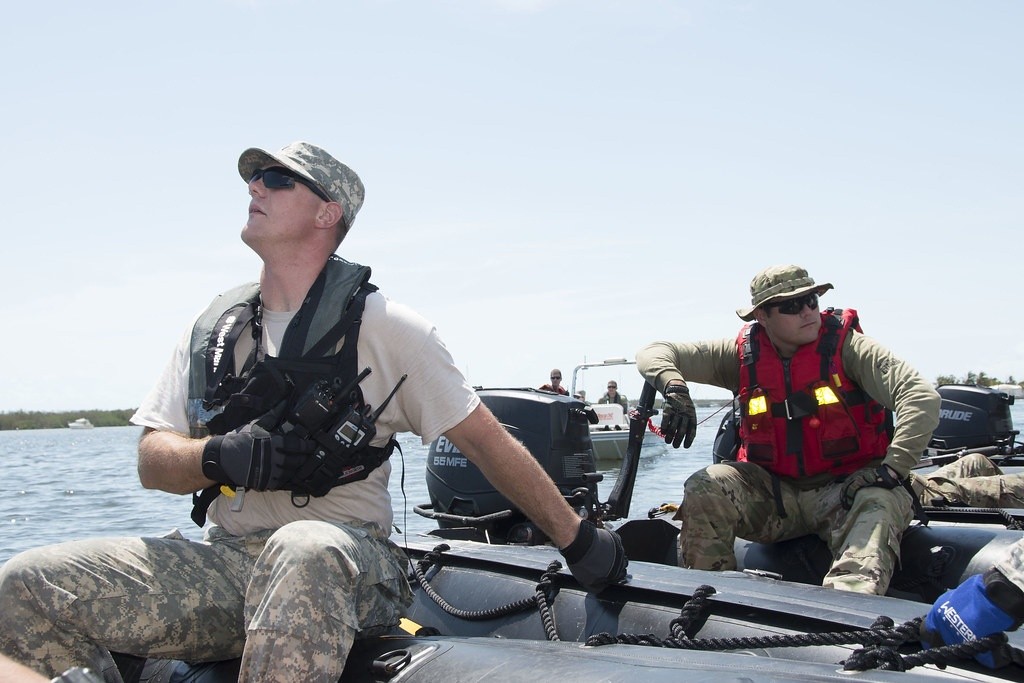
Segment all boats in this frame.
[68,418,94,430]
[568,356,670,462]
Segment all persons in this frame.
[597,380,631,417]
[633,265,943,597]
[0,140,628,682]
[538,368,570,397]
[876,451,1024,512]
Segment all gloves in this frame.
[839,464,902,511]
[558,519,628,593]
[661,385,697,449]
[918,567,1024,670]
[201,400,321,493]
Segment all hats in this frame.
[238,140,365,233]
[736,264,834,322]
[573,390,585,396]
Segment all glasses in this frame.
[551,376,560,379]
[766,291,819,315]
[249,166,331,203]
[574,395,584,399]
[608,386,616,388]
